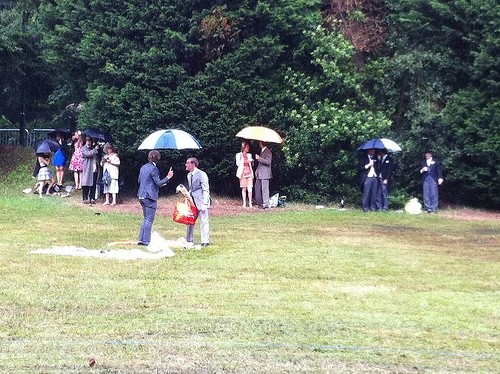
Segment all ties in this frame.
[379,158,382,166]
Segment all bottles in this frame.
[341,198,344,208]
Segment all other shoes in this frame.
[201,243,208,246]
[138,240,148,246]
[83,199,89,204]
[90,200,96,204]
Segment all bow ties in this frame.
[370,157,373,160]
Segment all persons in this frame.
[34,137,63,154]
[357,148,381,212]
[136,149,174,246]
[184,158,211,247]
[235,141,255,209]
[33,129,107,205]
[377,149,393,211]
[419,149,444,214]
[100,142,121,206]
[253,139,273,210]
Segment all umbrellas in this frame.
[137,127,203,172]
[356,138,403,158]
[234,126,284,158]
[80,128,116,149]
[48,127,74,139]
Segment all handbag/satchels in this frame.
[102,169,111,186]
[173,195,197,224]
[279,196,287,207]
